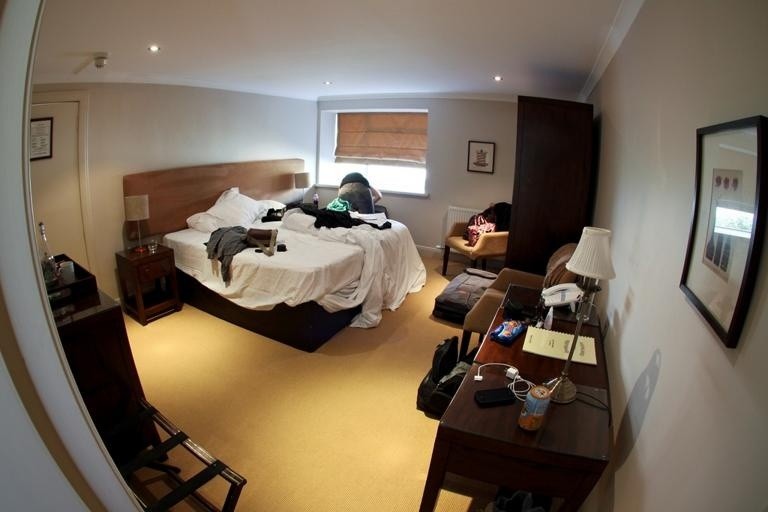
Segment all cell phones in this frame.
[474,386,516,409]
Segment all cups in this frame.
[59,261,75,284]
[148,240,157,251]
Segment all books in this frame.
[521,325,598,368]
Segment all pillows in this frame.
[257,200,286,211]
[186,186,270,233]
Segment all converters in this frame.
[507,367,520,379]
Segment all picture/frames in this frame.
[30,117,54,161]
[466,140,496,174]
[679,114,768,349]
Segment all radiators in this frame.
[445,205,485,247]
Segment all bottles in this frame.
[542,305,553,329]
[38,222,59,286]
[313,192,321,209]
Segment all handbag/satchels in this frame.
[417,336,479,419]
[467,223,496,246]
[247,229,278,249]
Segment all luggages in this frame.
[433,268,498,324]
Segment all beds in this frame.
[122,157,427,352]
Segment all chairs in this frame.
[460,242,581,365]
[442,201,509,278]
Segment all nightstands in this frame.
[115,243,181,327]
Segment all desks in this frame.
[40,253,248,512]
[419,285,615,512]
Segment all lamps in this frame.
[294,172,311,207]
[544,212,618,405]
[124,194,151,253]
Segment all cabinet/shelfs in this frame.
[507,91,598,275]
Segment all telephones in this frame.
[541,283,584,307]
